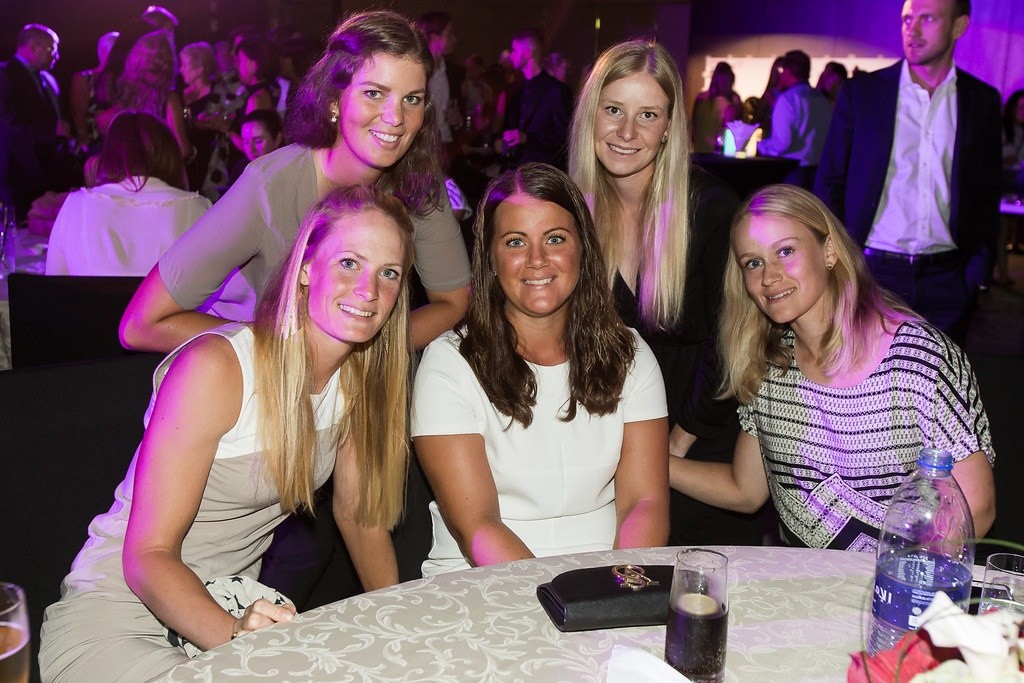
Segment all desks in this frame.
[693,150,801,196]
[140,547,1024,683]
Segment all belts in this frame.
[862,247,958,265]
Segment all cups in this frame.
[0,582,31,683]
[976,553,1024,616]
[664,547,728,682]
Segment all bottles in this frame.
[867,448,974,651]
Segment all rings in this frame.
[231,632,237,639]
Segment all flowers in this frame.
[848,590,1024,683]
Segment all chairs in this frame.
[7,271,146,370]
[0,352,166,683]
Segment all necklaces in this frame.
[319,149,335,192]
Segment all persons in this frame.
[0,0,872,319]
[38,183,410,683]
[669,184,997,559]
[410,162,669,583]
[815,0,1002,348]
[117,13,475,616]
[563,37,777,546]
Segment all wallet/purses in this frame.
[536,565,716,632]
